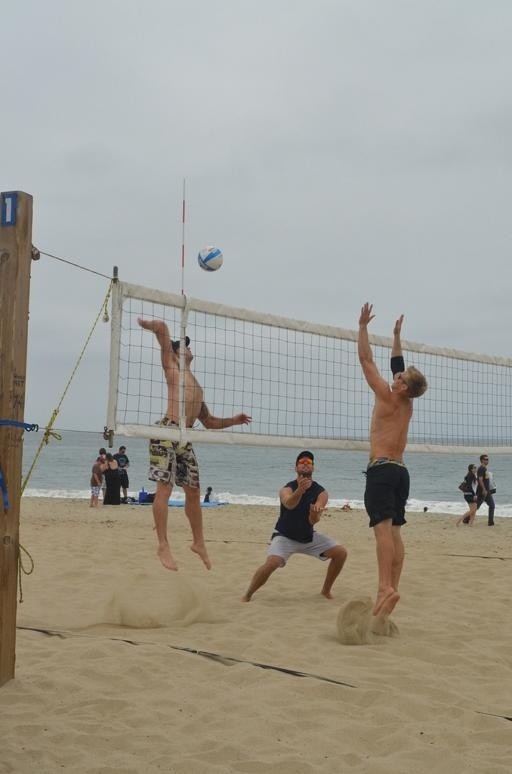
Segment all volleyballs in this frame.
[196,245,223,271]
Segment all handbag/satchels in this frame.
[458,479,472,492]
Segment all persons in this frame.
[454,462,479,528]
[462,454,498,528]
[90,456,106,507]
[240,450,348,603]
[357,303,430,629]
[204,486,217,503]
[103,453,121,506]
[99,448,108,499]
[137,314,254,572]
[112,445,129,503]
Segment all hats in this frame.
[296,451,314,466]
[171,336,190,351]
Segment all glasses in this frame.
[483,458,489,461]
[297,458,313,464]
[398,372,410,390]
